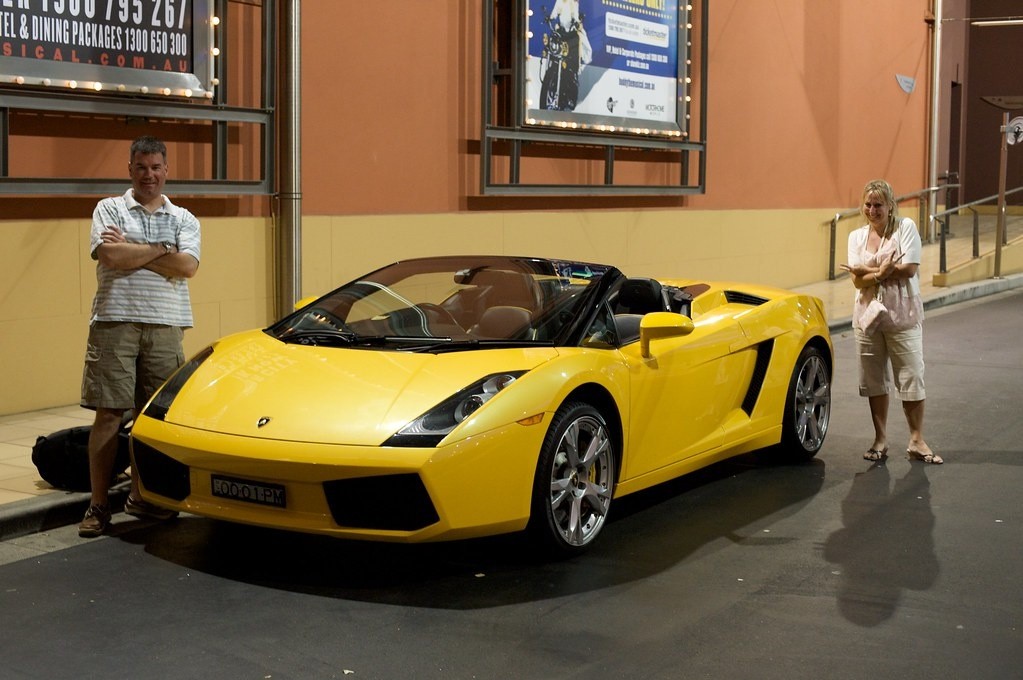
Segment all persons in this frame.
[839,180,943,465]
[77,135,202,537]
[546,0,592,75]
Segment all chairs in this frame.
[463,278,665,345]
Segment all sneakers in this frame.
[125,496,179,520]
[78,504,112,536]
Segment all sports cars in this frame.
[128,253,837,558]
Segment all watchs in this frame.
[160,241,172,255]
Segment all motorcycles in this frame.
[538,4,587,113]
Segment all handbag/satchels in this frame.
[859,284,888,336]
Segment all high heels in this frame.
[863,447,888,461]
[908,449,943,464]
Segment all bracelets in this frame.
[874,272,882,283]
